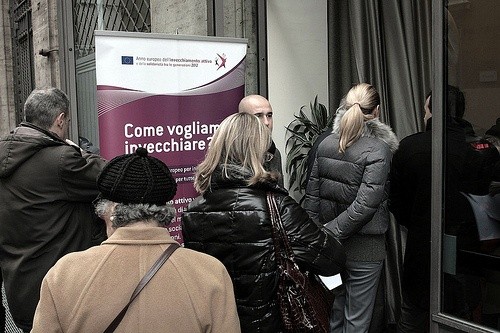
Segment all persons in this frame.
[180,112,347,333]
[475,117,500,196]
[238,95,284,188]
[0,85,111,333]
[306,97,387,333]
[29,147,241,333]
[390,85,493,333]
[304,83,400,333]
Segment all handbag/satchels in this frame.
[464,191,500,240]
[266,191,335,333]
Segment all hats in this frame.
[92,147,177,205]
